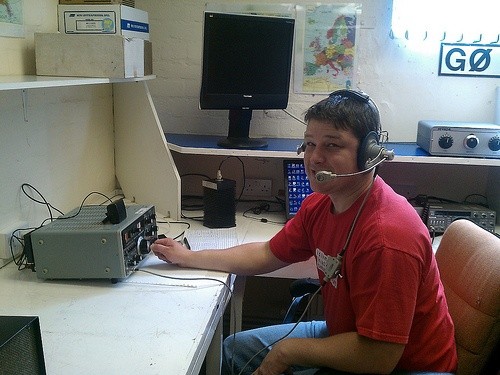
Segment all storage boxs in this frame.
[34,4,151,79]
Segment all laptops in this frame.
[283,160,314,220]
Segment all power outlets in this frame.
[242,177,272,197]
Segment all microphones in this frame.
[316,158,386,183]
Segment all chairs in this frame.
[281,217,500,375]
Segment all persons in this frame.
[150,90,459,375]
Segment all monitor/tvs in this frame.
[197,10,295,148]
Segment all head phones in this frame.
[295,90,395,175]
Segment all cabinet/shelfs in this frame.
[0,73,500,375]
